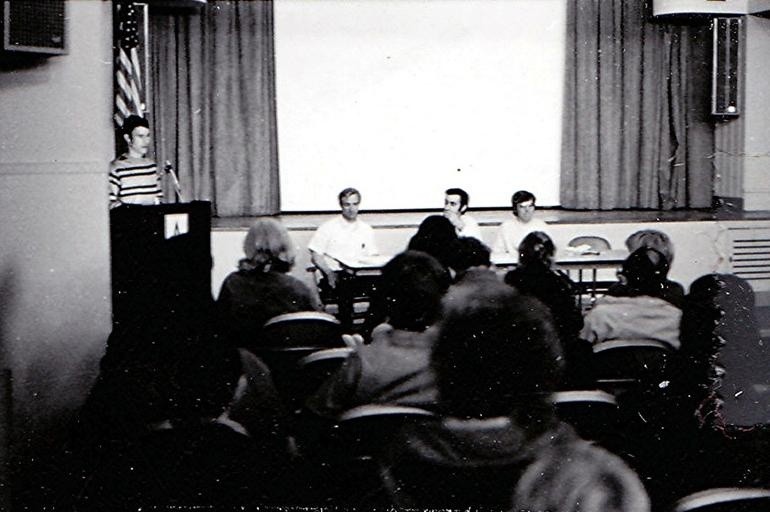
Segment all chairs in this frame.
[260,312,355,401]
[307,267,370,321]
[593,339,675,385]
[568,236,612,313]
[334,406,440,460]
[407,454,534,511]
[546,390,619,440]
[672,486,770,512]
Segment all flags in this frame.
[112,0,147,156]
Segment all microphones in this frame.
[163,159,182,193]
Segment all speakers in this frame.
[0,1,68,57]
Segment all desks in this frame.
[336,250,628,334]
